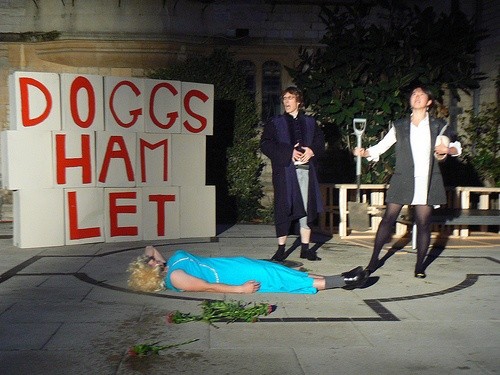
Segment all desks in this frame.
[381,206,500,227]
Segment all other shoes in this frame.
[300,249,321,261]
[271,250,286,262]
[340,271,369,290]
[415,272,425,278]
[364,259,380,271]
[341,266,363,279]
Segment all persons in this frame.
[259,87,326,261]
[354,85,463,279]
[126,244,369,295]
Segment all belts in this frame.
[302,162,309,165]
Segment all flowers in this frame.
[164,299,272,330]
[126,338,199,359]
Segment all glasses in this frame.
[283,96,296,100]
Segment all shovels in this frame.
[348,119,369,231]
